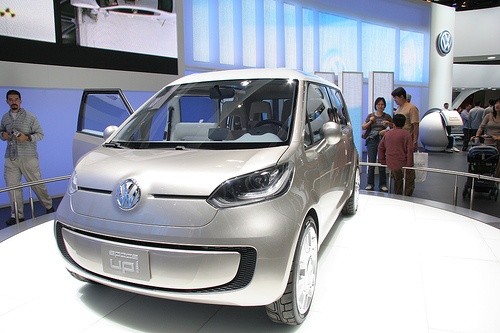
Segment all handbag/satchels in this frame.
[361,112,374,139]
[413,148,428,183]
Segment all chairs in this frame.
[221,98,329,146]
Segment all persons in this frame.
[444,103,463,114]
[381,87,419,152]
[0,90,54,226]
[472,98,500,148]
[461,103,473,154]
[482,99,497,120]
[362,97,393,192]
[468,99,484,144]
[377,114,416,196]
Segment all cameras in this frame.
[10,128,20,137]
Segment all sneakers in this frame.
[381,185,388,191]
[364,184,373,190]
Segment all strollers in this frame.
[462,135,500,201]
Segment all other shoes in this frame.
[45,205,54,214]
[6,217,26,226]
[442,147,452,153]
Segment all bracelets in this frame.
[25,134,32,141]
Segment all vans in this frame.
[54,67,360,325]
